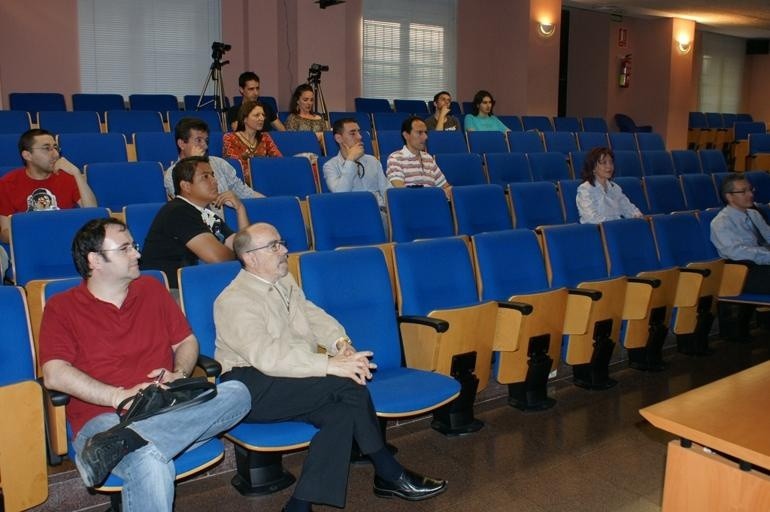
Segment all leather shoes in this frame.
[373,467,447,500]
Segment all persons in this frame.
[465,90,511,134]
[285,84,328,144]
[140,156,249,288]
[39,218,252,512]
[424,91,460,132]
[212,221,449,510]
[221,102,283,179]
[711,174,770,295]
[386,118,451,198]
[163,117,266,205]
[229,72,284,131]
[323,118,394,225]
[0,129,98,243]
[575,147,643,224]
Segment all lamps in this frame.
[537,23,555,38]
[677,41,690,55]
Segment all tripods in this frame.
[315,84,328,121]
[195,60,229,133]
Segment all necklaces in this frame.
[239,132,257,149]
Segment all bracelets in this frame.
[174,369,192,379]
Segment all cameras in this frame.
[308,63,329,84]
[211,42,232,59]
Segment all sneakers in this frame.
[75,433,126,488]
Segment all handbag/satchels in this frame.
[121,377,217,422]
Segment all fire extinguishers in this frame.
[619,52,633,88]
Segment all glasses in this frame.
[104,243,140,252]
[31,145,59,152]
[725,186,755,193]
[245,239,287,253]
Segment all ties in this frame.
[745,213,770,249]
